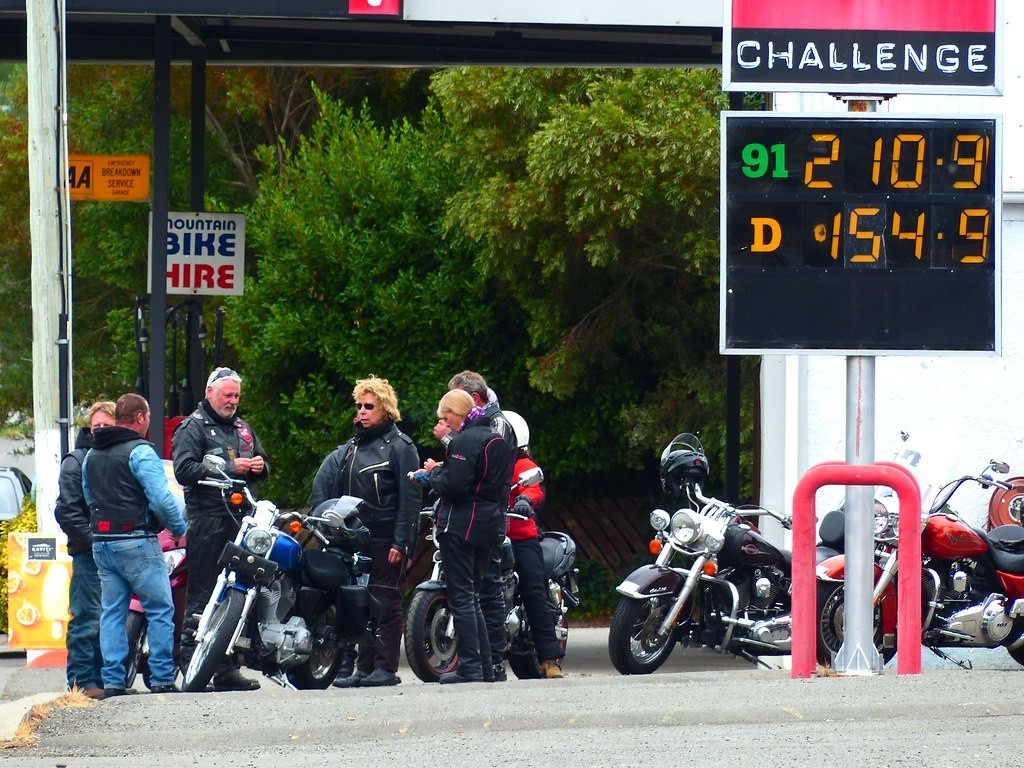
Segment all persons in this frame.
[81,393,188,695]
[170,366,274,691]
[409,368,566,682]
[53,400,117,700]
[303,373,421,686]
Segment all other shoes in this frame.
[205,681,213,692]
[539,657,564,678]
[493,661,507,681]
[214,669,261,693]
[438,667,483,685]
[151,684,182,694]
[484,675,495,682]
[72,684,106,700]
[102,688,139,696]
[333,668,368,689]
[359,666,401,687]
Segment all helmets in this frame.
[311,494,372,551]
[502,409,530,447]
[659,450,709,498]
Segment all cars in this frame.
[0,467,32,523]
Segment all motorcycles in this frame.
[608,430,1024,675]
[125,454,382,693]
[406,467,580,685]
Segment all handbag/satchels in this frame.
[216,540,281,590]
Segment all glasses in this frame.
[212,369,241,383]
[354,402,380,410]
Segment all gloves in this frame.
[513,494,534,517]
